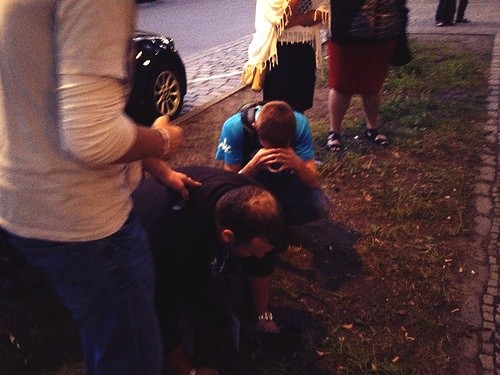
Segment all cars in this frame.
[125,31,187,124]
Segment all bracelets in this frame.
[153,127,170,159]
[258,312,273,320]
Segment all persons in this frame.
[436,0,456,27]
[248,0,331,114]
[0,0,202,375]
[137,165,280,375]
[214,100,331,226]
[326,0,404,152]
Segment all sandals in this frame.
[326,132,343,152]
[364,128,391,147]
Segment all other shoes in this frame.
[437,20,470,27]
